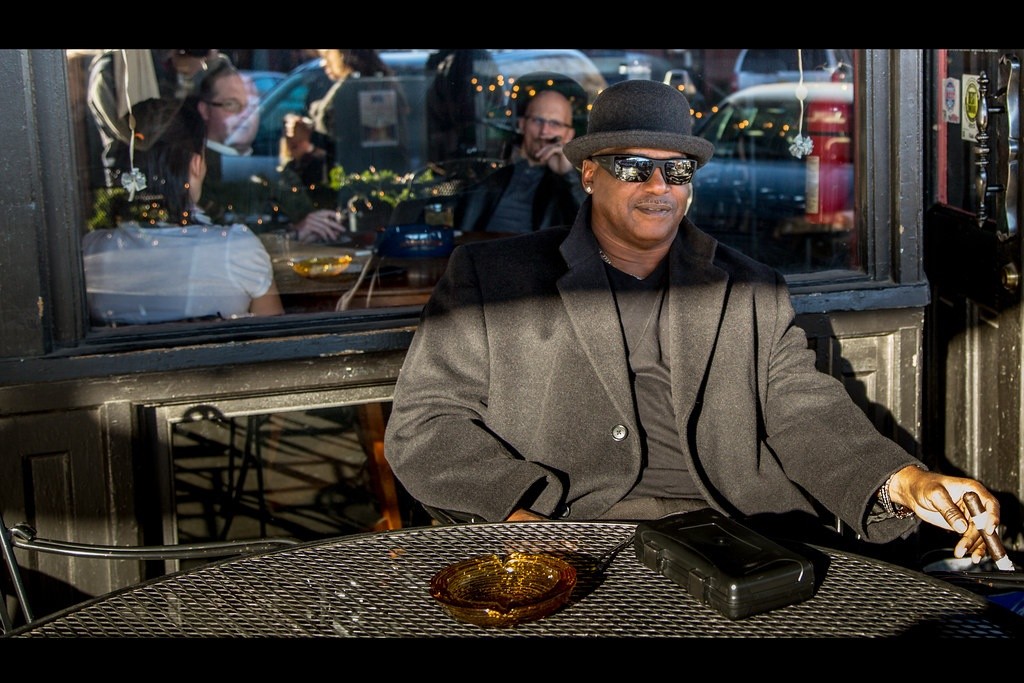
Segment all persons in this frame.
[456,90,592,251]
[81,98,288,325]
[380,79,1002,565]
[197,69,348,245]
[89,45,509,227]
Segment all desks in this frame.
[0,234,1024,683]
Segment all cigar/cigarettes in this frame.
[548,135,561,144]
[963,492,1015,571]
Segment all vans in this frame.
[726,47,855,100]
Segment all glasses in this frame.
[529,116,572,129]
[207,99,264,115]
[586,154,697,185]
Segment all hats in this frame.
[562,80,715,170]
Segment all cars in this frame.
[592,50,710,117]
[210,67,315,152]
[209,49,612,224]
[686,80,855,261]
